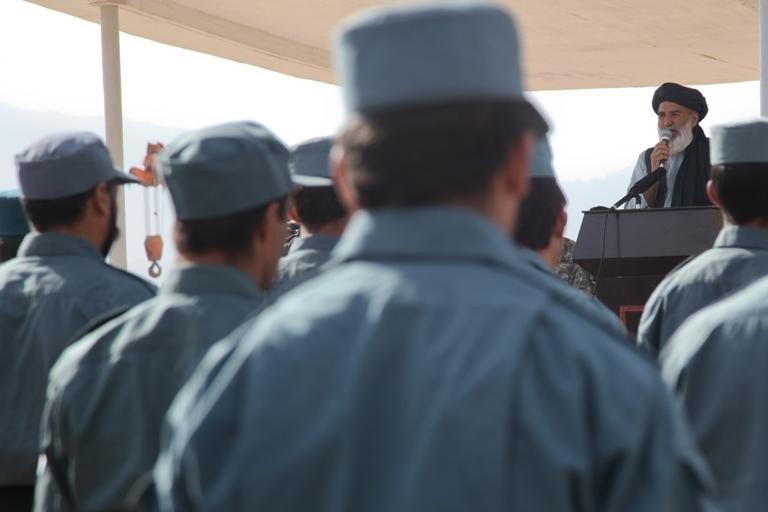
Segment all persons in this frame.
[148,0,719,511]
[507,113,569,275]
[268,133,345,303]
[34,119,305,511]
[623,82,713,207]
[0,124,161,510]
[635,117,767,448]
[0,187,30,266]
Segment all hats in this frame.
[159,120,333,220]
[652,83,708,122]
[290,137,333,181]
[530,134,554,176]
[341,6,550,135]
[1,190,30,236]
[14,131,142,201]
[709,121,768,166]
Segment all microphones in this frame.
[659,129,673,167]
[609,168,666,212]
